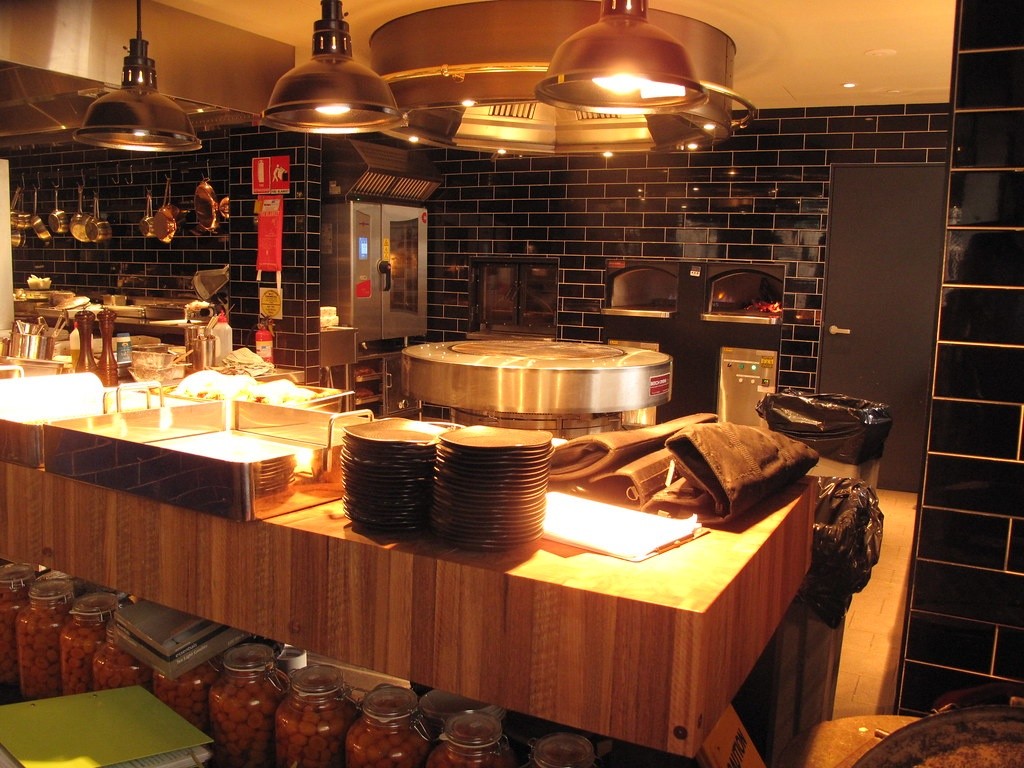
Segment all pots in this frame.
[9,187,31,249]
[48,186,69,233]
[31,188,52,242]
[142,179,181,243]
[194,178,221,234]
[69,188,112,242]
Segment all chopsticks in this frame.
[207,314,220,329]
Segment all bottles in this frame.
[70,307,118,386]
[427,710,595,768]
[274,665,354,768]
[209,644,290,768]
[345,683,434,768]
[60,594,217,729]
[213,311,233,367]
[0,563,74,701]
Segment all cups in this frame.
[185,327,215,369]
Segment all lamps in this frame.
[72,1,203,152]
[533,1,709,114]
[261,0,402,134]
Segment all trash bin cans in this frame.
[732,476,873,768]
[762,391,892,496]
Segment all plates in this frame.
[340,418,448,530]
[431,425,554,551]
[420,689,508,722]
[258,454,296,491]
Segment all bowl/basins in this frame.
[27,280,73,307]
[103,295,127,306]
[129,344,192,385]
